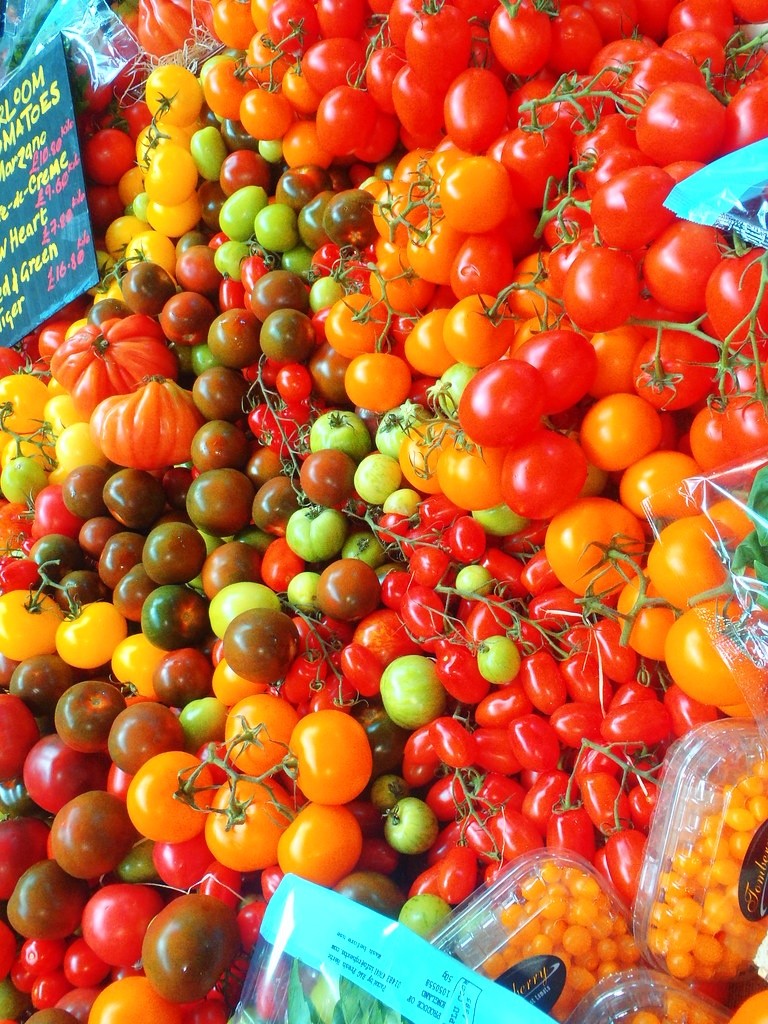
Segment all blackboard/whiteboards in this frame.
[0,30,101,349]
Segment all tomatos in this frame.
[2,0,768,1024]
